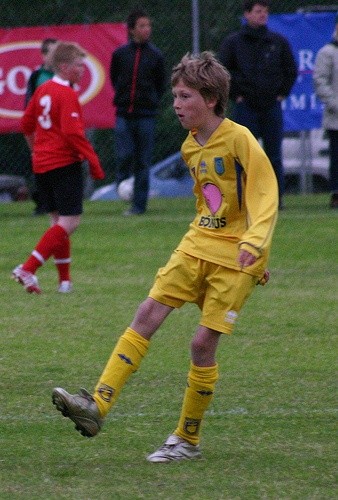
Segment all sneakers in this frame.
[147,433,203,465]
[12,264,43,295]
[52,386,102,438]
[56,281,75,296]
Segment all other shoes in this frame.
[122,207,142,216]
[331,194,338,209]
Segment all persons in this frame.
[11,40,105,294]
[50,51,280,466]
[312,13,338,211]
[215,0,300,213]
[108,9,174,221]
[24,37,61,216]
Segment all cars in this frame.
[90,137,332,198]
